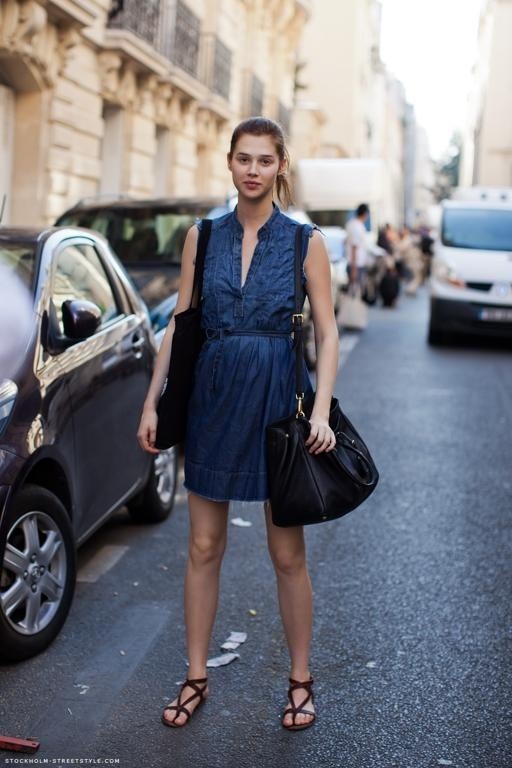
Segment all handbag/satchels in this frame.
[264,391,379,528]
[152,218,214,452]
[335,293,369,331]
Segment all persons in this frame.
[136,117,339,733]
[344,203,439,307]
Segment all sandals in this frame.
[161,677,209,727]
[280,674,318,732]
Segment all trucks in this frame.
[283,150,399,293]
[417,184,509,350]
